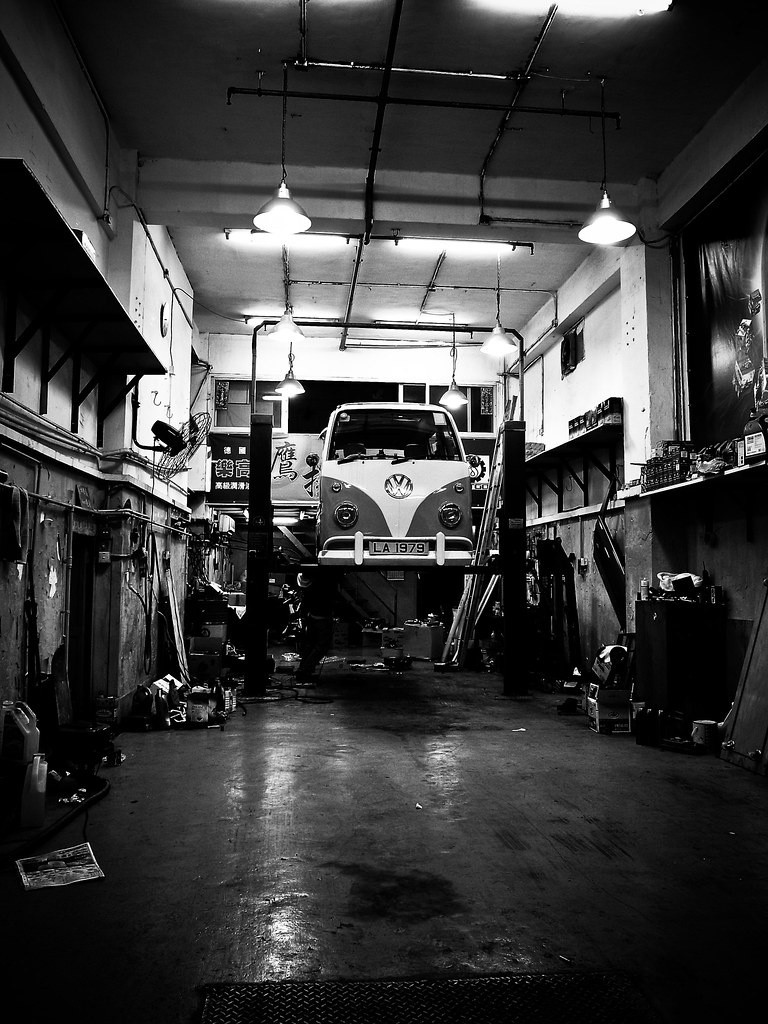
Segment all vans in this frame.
[306,402,475,569]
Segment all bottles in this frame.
[641,578,649,601]
[213,677,225,713]
[643,447,698,492]
[0,700,40,763]
[744,412,766,463]
[20,753,48,828]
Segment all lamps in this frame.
[267,245,306,343]
[439,313,469,410]
[481,260,517,352]
[275,342,305,394]
[253,69,312,234]
[578,85,637,245]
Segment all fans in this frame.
[131,412,213,481]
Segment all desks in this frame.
[0,774,111,848]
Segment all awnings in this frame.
[0,157,168,419]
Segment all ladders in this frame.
[451,394,518,667]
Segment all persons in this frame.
[297,568,341,688]
